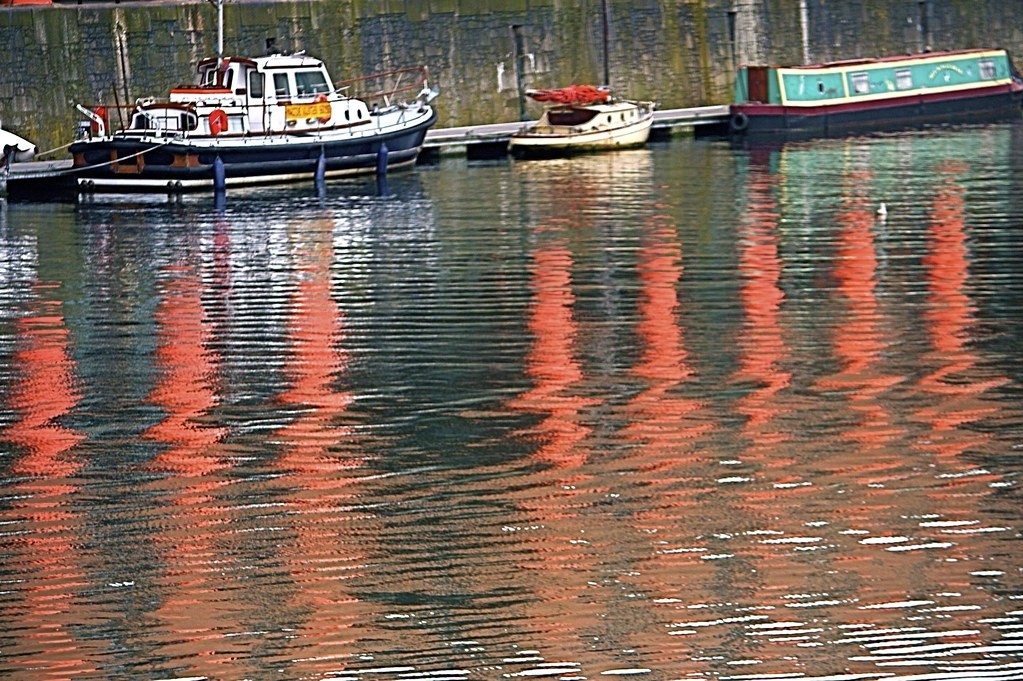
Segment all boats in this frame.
[729,46,1023,136]
[67,0,437,194]
[506,99,656,157]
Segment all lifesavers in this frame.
[314,94,332,123]
[93,106,109,132]
[731,113,749,130]
[209,109,228,136]
